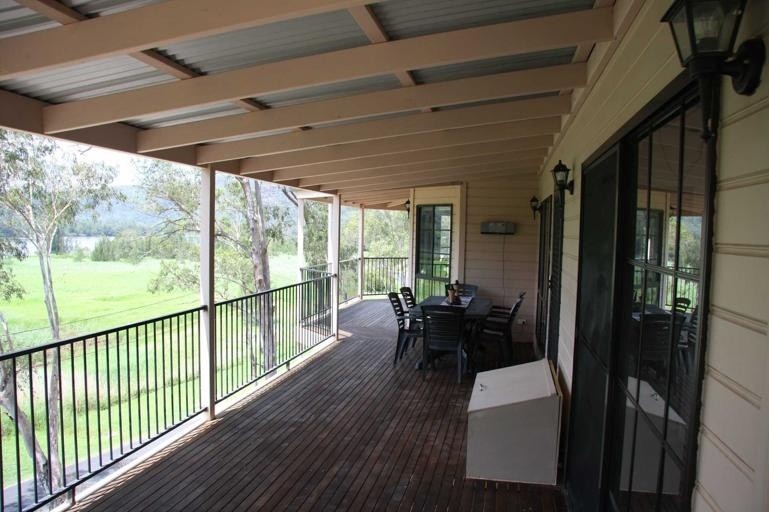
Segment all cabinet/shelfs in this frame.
[620,376,688,497]
[465,356,564,487]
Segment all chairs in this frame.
[420,305,470,384]
[491,289,526,320]
[445,283,476,298]
[470,297,523,376]
[400,287,426,346]
[388,292,426,365]
[627,286,697,400]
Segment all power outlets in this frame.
[517,319,527,327]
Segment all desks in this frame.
[408,295,493,372]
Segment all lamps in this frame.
[530,195,543,220]
[480,222,515,235]
[660,1,766,139]
[550,159,574,207]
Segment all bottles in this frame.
[448,280,460,303]
